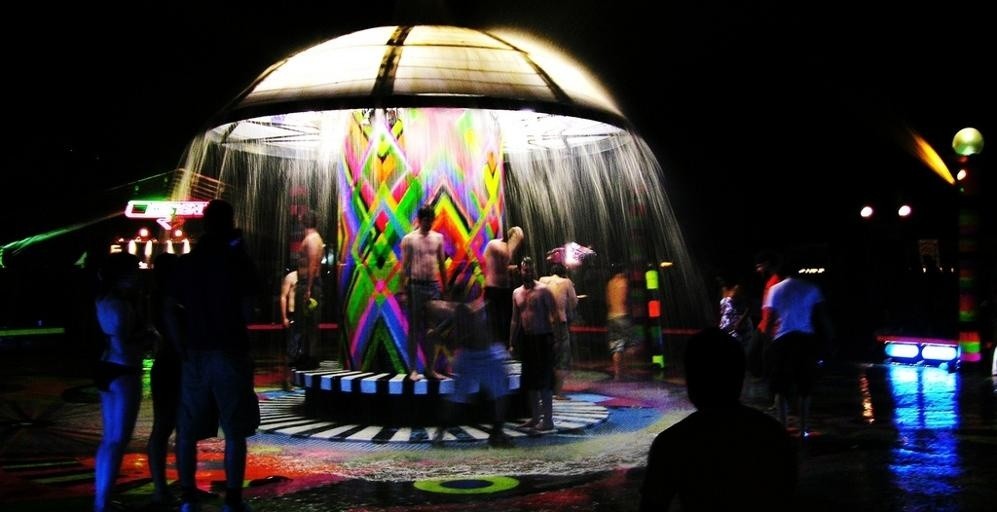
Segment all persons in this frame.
[606,258,645,387]
[540,259,578,396]
[84,249,149,512]
[297,208,324,372]
[508,255,565,439]
[481,225,524,344]
[637,258,834,510]
[395,204,447,385]
[172,198,262,512]
[425,285,514,444]
[138,250,223,512]
[279,267,300,394]
